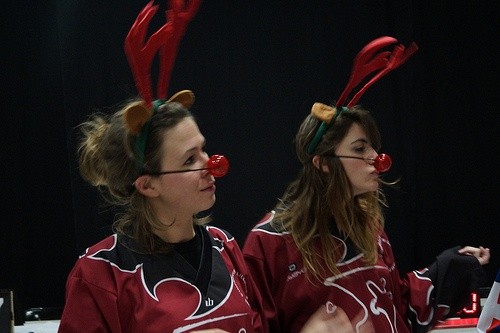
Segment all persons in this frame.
[242,100,491,333]
[57,90,355,333]
